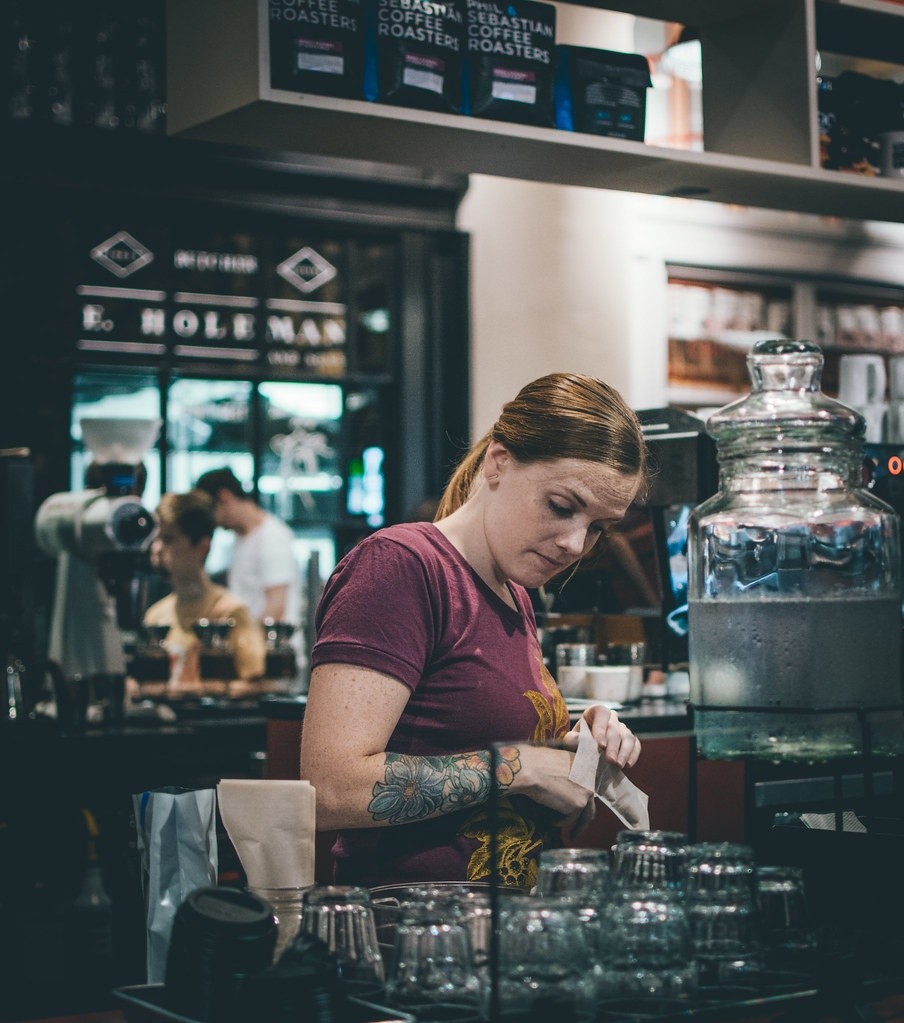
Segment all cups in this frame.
[758,865,811,950]
[390,885,492,992]
[166,886,279,1023]
[298,886,387,995]
[555,642,596,698]
[497,830,759,998]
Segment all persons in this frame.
[543,532,661,645]
[190,469,308,667]
[302,374,647,886]
[123,491,267,711]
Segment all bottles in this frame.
[261,621,298,681]
[134,627,169,685]
[686,339,903,764]
[190,618,235,684]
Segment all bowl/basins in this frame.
[585,665,642,700]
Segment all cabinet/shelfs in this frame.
[166,0,904,224]
[665,262,904,511]
